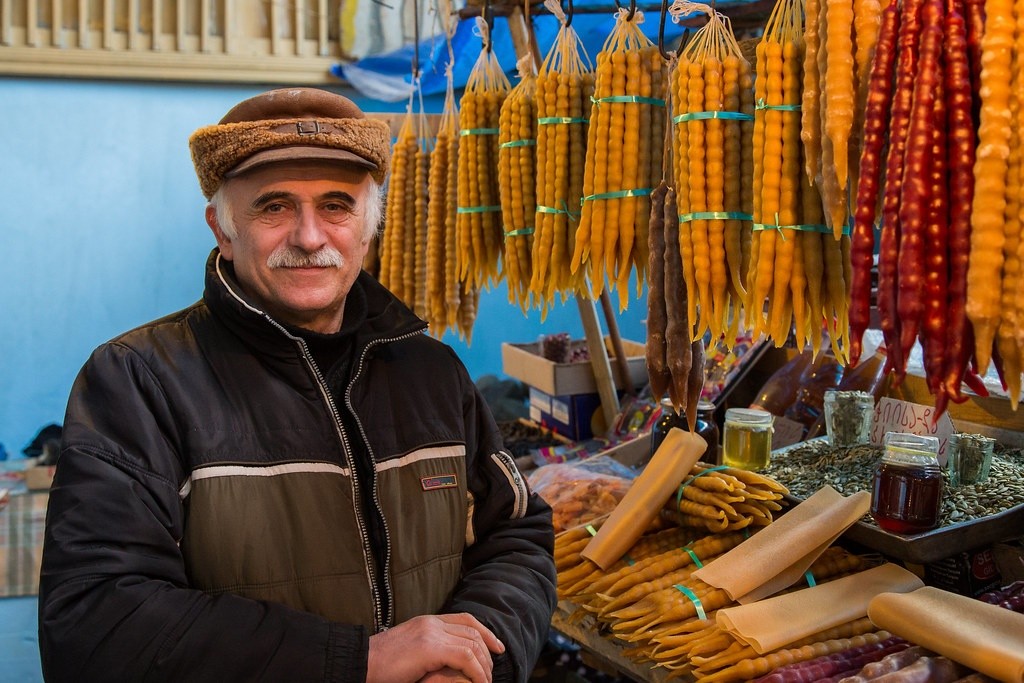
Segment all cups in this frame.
[948,434,994,486]
[824,395,874,447]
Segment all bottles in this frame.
[870,432,941,534]
[722,408,772,472]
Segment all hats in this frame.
[188,87,391,200]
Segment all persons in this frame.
[41,85,559,683]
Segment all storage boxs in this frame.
[0,439,57,600]
[502,336,1024,566]
